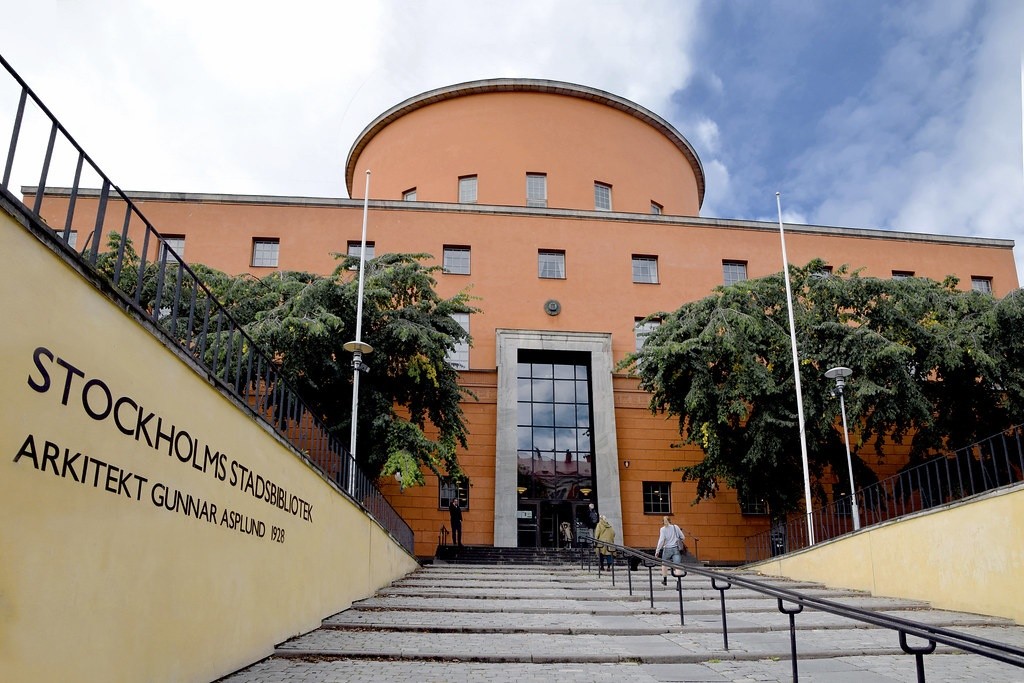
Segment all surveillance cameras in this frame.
[625,461,628,468]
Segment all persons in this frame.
[560,524,573,549]
[449,498,464,547]
[594,515,615,572]
[655,516,685,586]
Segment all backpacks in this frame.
[589,510,598,524]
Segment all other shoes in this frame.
[458,543,464,546]
[661,582,667,585]
[601,568,604,570]
[453,543,457,545]
[607,567,610,571]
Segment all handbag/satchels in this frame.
[593,537,599,548]
[673,524,686,555]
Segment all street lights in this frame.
[823,366,862,530]
[341,340,373,495]
